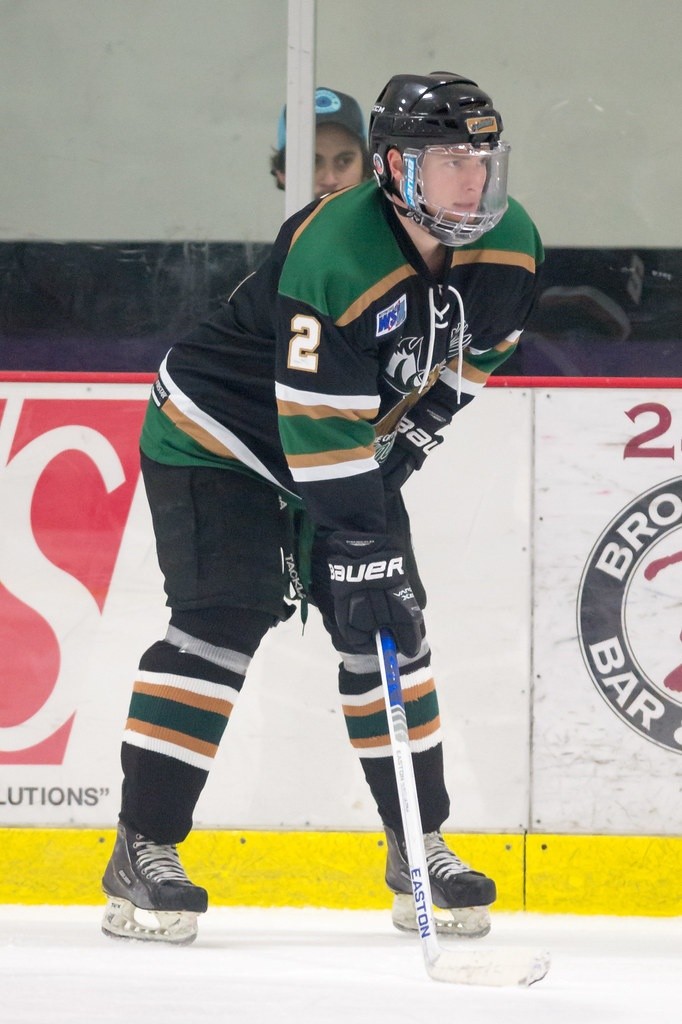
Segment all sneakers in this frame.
[382,824,497,939]
[101,820,208,945]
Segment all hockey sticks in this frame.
[375,625,556,986]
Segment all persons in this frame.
[265,91,368,197]
[103,72,557,944]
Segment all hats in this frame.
[277,87,364,150]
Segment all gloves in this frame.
[381,399,453,502]
[323,523,427,659]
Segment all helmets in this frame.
[368,70,511,247]
